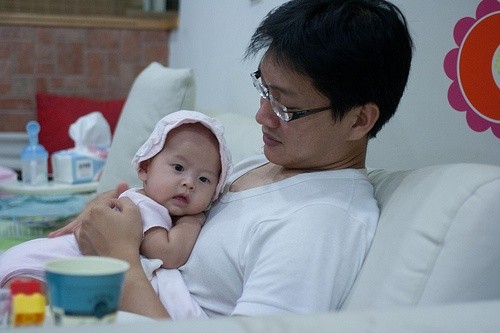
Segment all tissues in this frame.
[51,110,113,182]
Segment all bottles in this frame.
[19,122,48,187]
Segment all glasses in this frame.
[250,69,333,121]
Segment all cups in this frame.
[44,255,130,323]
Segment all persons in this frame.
[0,109,231,306]
[47,0,414,322]
